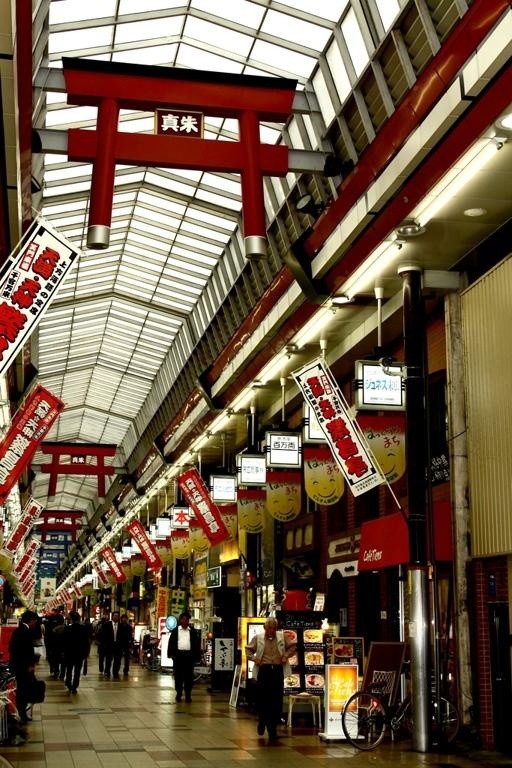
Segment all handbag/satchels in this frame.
[27,670,46,704]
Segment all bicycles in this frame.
[193,648,212,682]
[143,636,162,671]
[341,660,462,751]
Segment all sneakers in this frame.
[256,723,280,744]
[175,694,182,701]
[184,696,192,703]
[51,670,79,695]
[98,669,130,678]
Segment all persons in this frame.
[244,617,295,739]
[168,612,199,703]
[8,607,134,722]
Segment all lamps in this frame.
[296,193,325,220]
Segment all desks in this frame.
[287,695,322,729]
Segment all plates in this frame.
[297,694,313,697]
